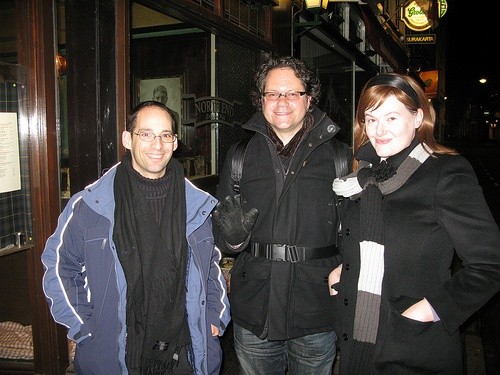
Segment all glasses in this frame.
[262,91,307,100]
[134,131,177,143]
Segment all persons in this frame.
[41,101,232,375]
[328,72,500,375]
[211,57,356,375]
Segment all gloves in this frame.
[213,194,260,246]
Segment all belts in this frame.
[245,242,339,263]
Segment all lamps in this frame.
[291,0,328,58]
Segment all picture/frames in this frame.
[135,74,186,147]
